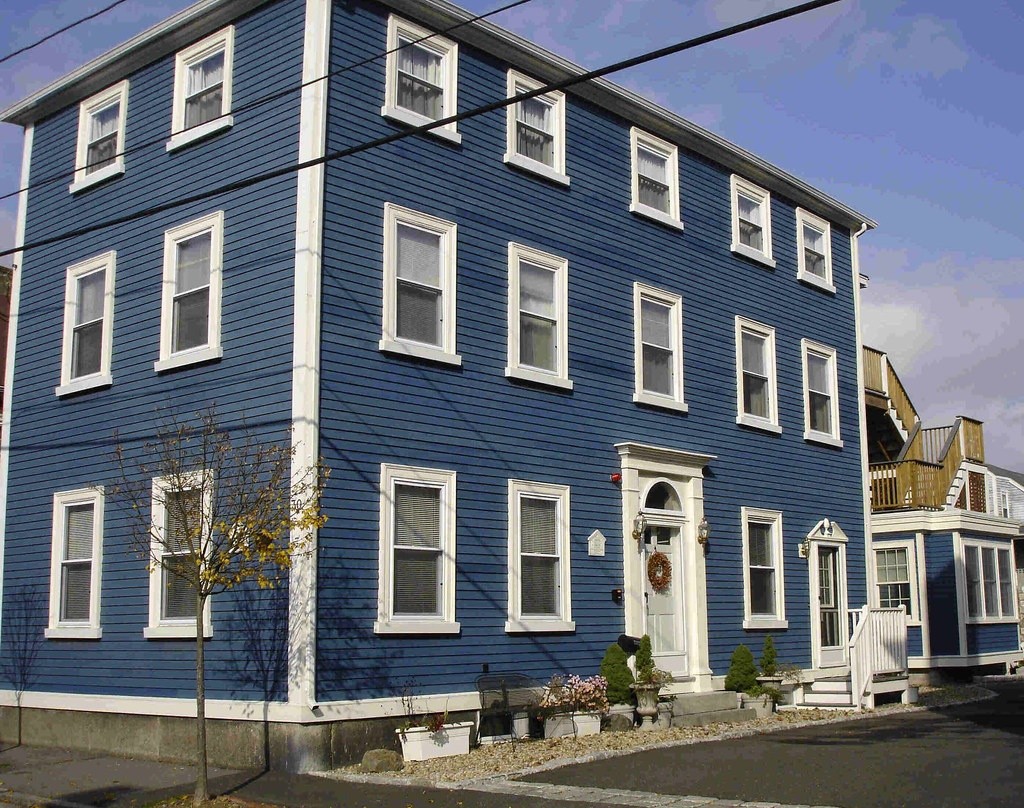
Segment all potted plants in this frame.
[630,634,679,732]
[599,643,638,730]
[724,636,801,718]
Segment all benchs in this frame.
[473,673,578,753]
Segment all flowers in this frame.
[649,551,673,589]
[534,671,609,722]
[394,676,453,742]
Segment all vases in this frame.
[395,721,474,762]
[544,709,602,739]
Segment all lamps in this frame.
[697,517,712,544]
[633,508,647,545]
[799,536,811,558]
